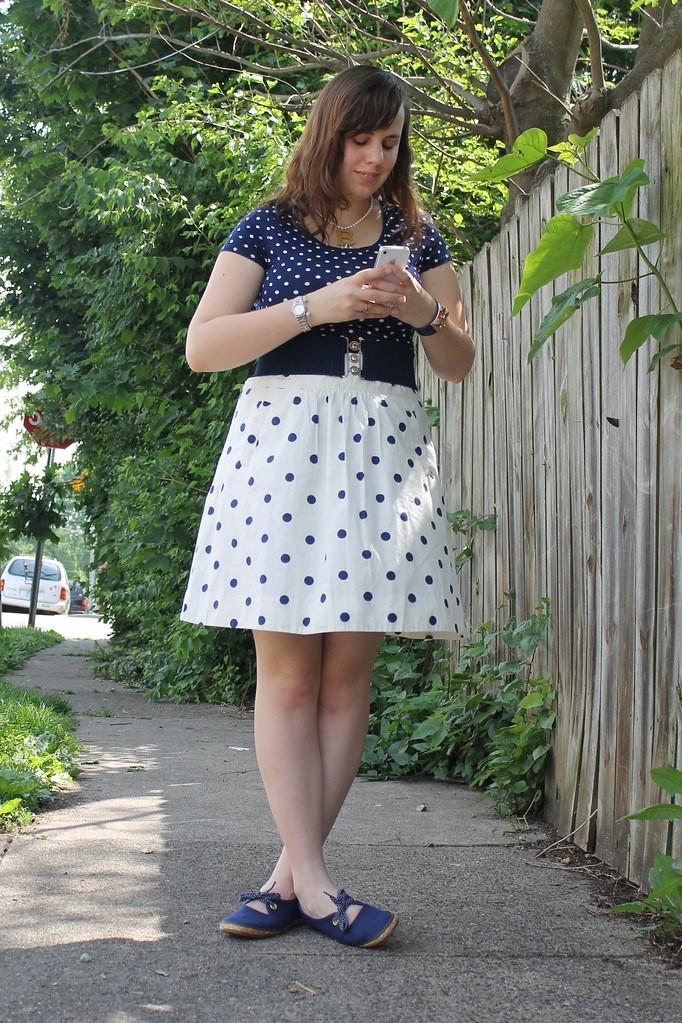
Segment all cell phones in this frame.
[375,245,410,269]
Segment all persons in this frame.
[181,65,475,947]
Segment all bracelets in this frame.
[414,301,449,336]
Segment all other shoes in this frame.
[297,886,398,947]
[220,881,306,936]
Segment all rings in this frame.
[363,302,367,313]
[390,303,395,308]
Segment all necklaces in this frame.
[314,196,373,246]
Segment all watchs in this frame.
[292,296,311,333]
